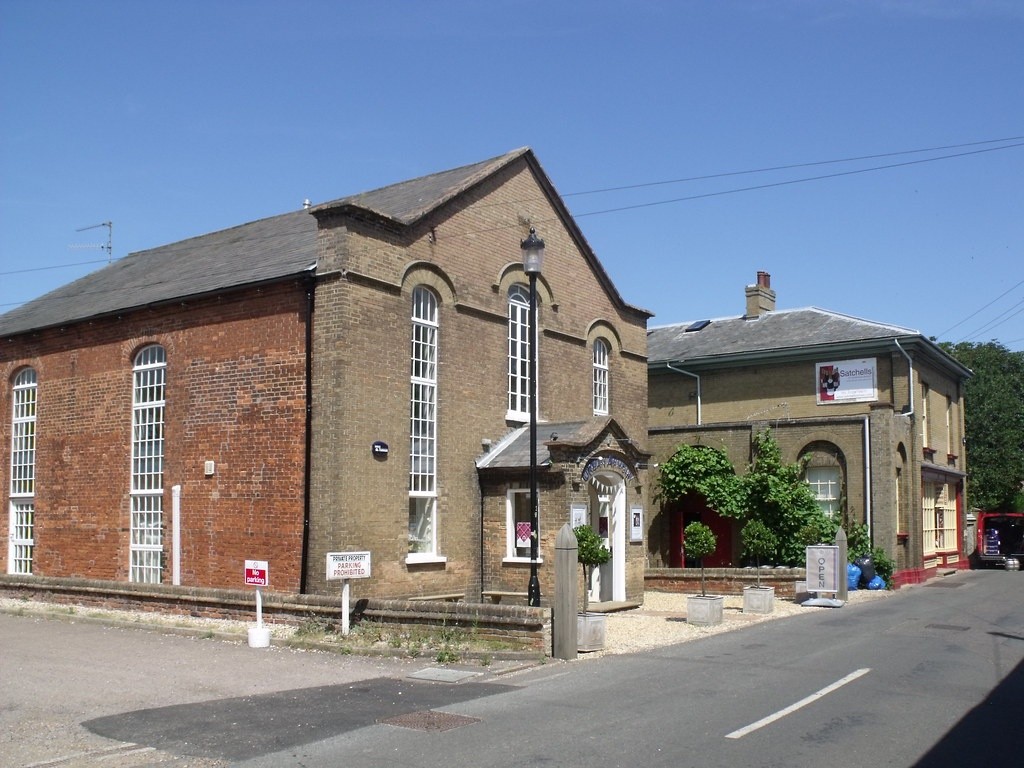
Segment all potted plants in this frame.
[742,518,779,616]
[571,523,613,652]
[682,521,726,627]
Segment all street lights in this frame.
[520,227,545,607]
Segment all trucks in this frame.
[978,510,1024,568]
